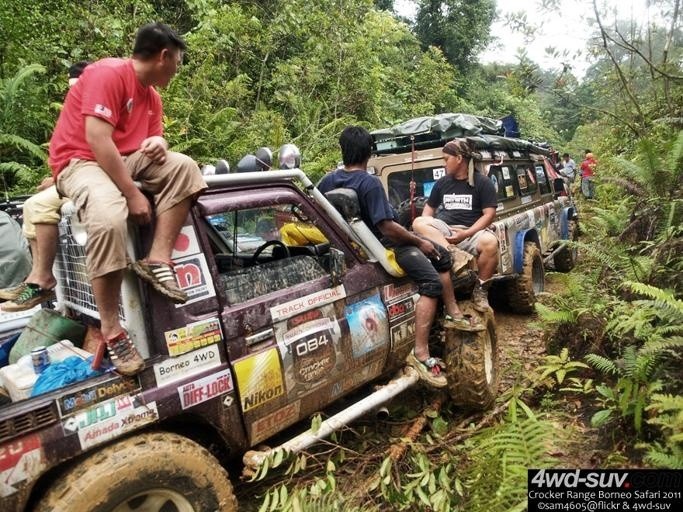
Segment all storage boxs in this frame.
[0,339,94,403]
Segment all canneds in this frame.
[30,346,52,375]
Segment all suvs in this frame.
[348,108,584,308]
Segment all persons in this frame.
[315,125,487,388]
[0,64,88,311]
[411,140,499,313]
[577,150,590,193]
[254,218,328,250]
[562,153,576,197]
[45,21,208,376]
[580,152,597,200]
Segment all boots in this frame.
[445,243,474,275]
[472,276,497,313]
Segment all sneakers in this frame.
[406,345,448,389]
[440,314,486,333]
[0,282,57,311]
[105,330,145,376]
[132,258,189,304]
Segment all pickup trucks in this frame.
[2,141,510,512]
